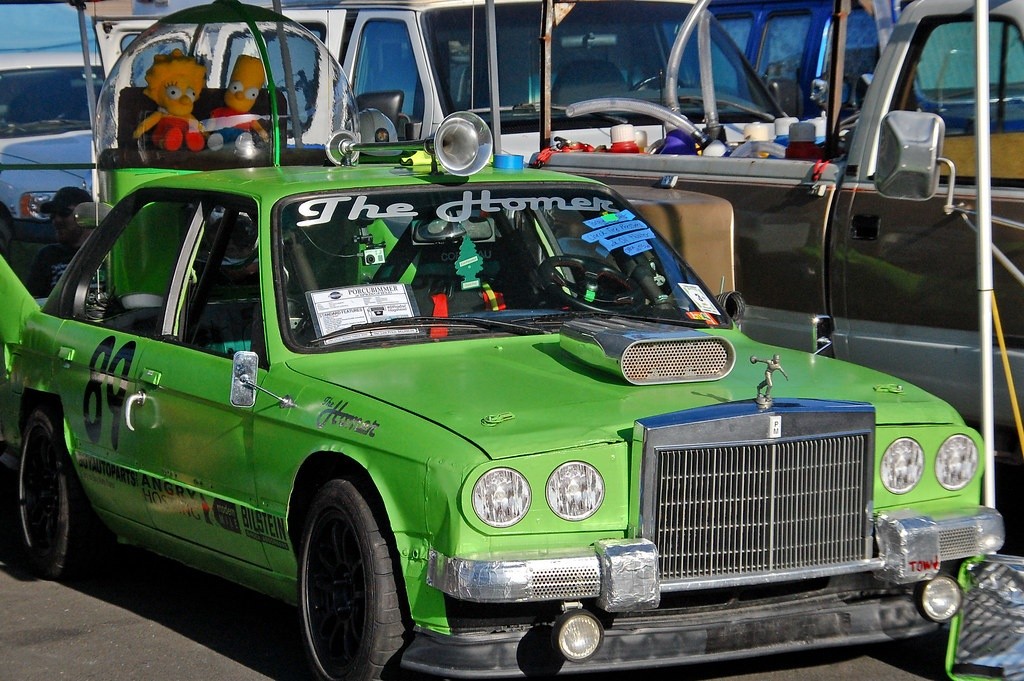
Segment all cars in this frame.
[0,0,1009,681]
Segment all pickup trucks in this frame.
[529,0,1024,468]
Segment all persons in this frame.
[199,54,269,154]
[133,48,208,152]
[23,187,107,299]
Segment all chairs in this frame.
[552,59,625,104]
